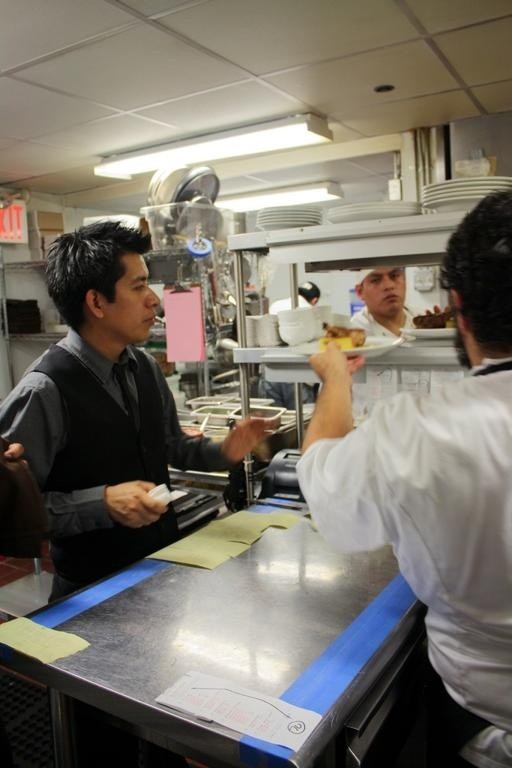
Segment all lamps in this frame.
[92,113,334,180]
[214,181,345,213]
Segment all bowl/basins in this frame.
[278,306,333,346]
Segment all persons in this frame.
[0,221,282,606]
[295,189,511,767]
[0,443,28,482]
[264,265,416,410]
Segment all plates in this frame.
[292,335,405,359]
[399,328,459,339]
[235,315,281,347]
[255,176,512,230]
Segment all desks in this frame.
[0,497,430,768]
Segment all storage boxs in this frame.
[139,201,230,250]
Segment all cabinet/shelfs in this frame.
[226,210,472,508]
[0,248,210,397]
